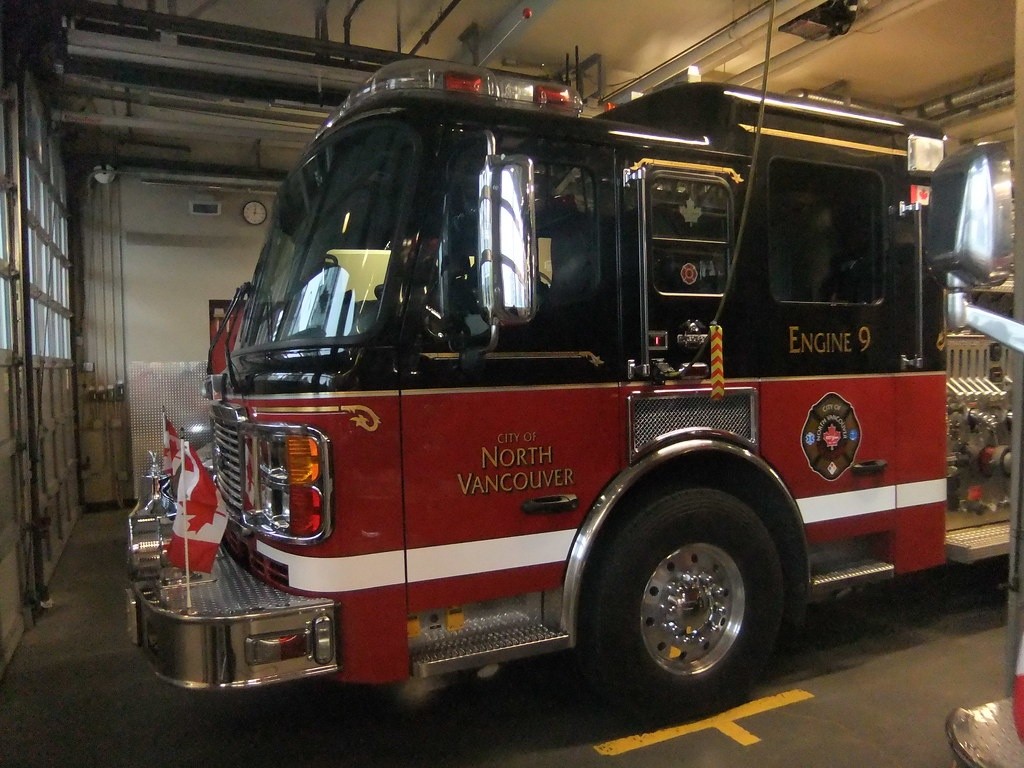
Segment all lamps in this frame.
[93,162,116,185]
[778,0,861,42]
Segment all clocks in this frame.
[243,200,268,225]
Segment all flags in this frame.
[164,418,182,476]
[164,442,228,573]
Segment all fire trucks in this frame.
[126,54,1024,728]
